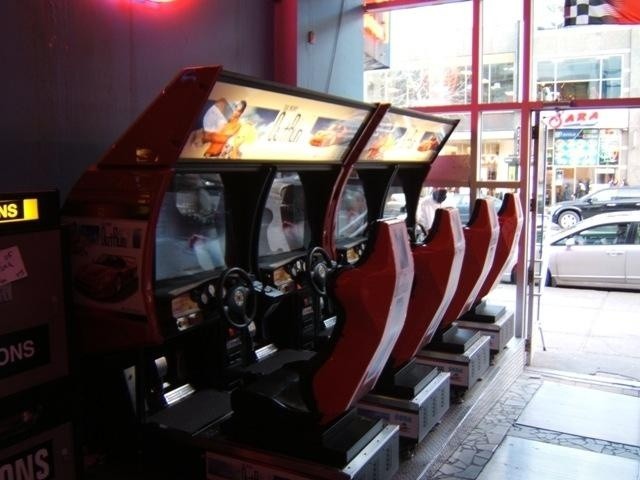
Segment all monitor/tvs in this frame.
[155,169,409,281]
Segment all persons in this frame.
[181,95,247,159]
[561,177,590,201]
[418,184,457,237]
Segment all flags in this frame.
[562,0,640,28]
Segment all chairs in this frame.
[229,211,416,466]
[374,202,466,403]
[460,192,528,324]
[423,198,503,355]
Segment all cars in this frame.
[380,187,640,292]
